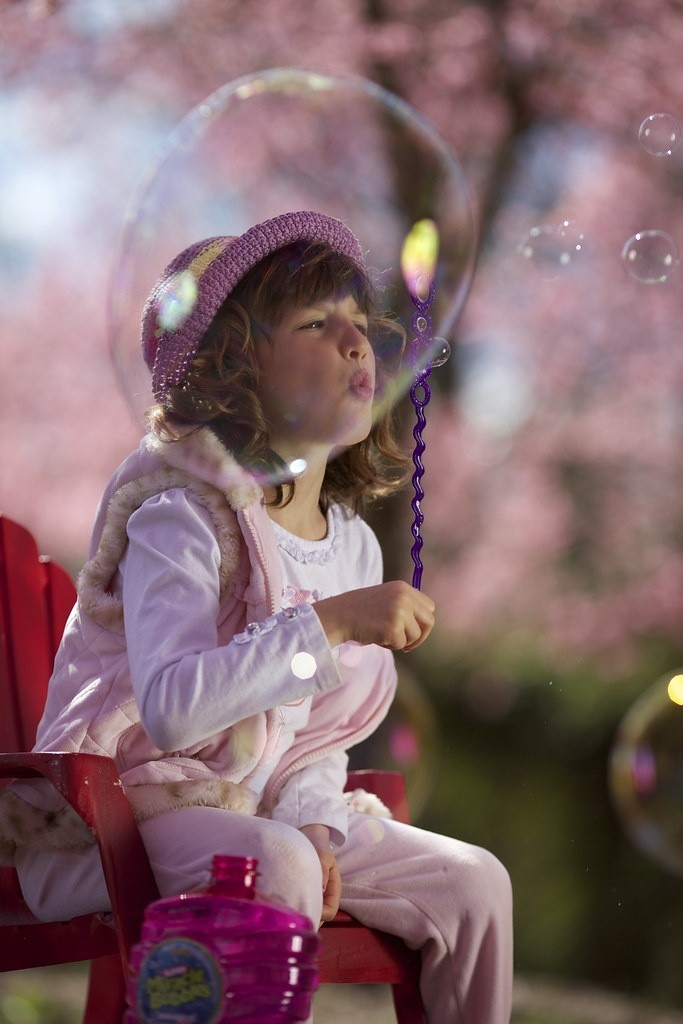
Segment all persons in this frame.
[1,209,515,1024]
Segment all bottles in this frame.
[129,852,320,1022]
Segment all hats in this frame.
[139,211,367,410]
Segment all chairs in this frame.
[0,517,424,1024]
